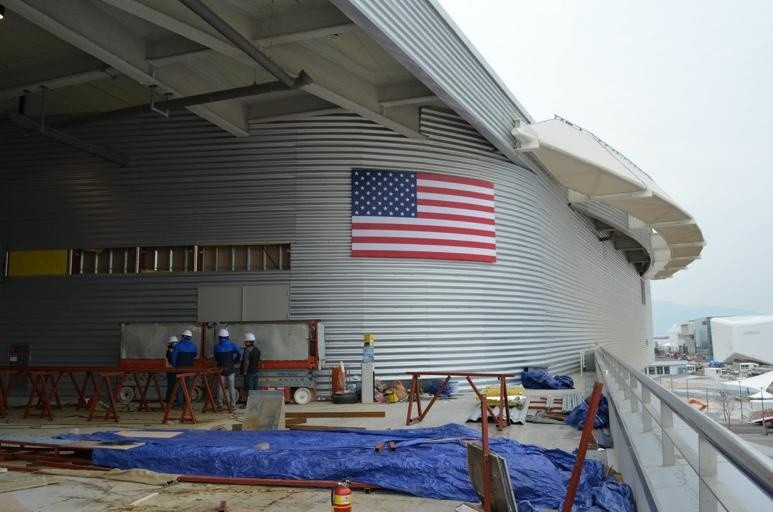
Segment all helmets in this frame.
[180,329,192,338]
[166,335,179,344]
[217,329,229,337]
[241,332,255,342]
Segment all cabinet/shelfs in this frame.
[0,356,234,427]
[404,370,515,432]
[4,240,292,277]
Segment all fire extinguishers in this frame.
[331,478,352,512]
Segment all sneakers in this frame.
[216,405,245,411]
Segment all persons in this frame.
[238,332,260,409]
[213,328,241,412]
[171,329,197,407]
[164,336,178,408]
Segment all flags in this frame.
[350,167,497,265]
